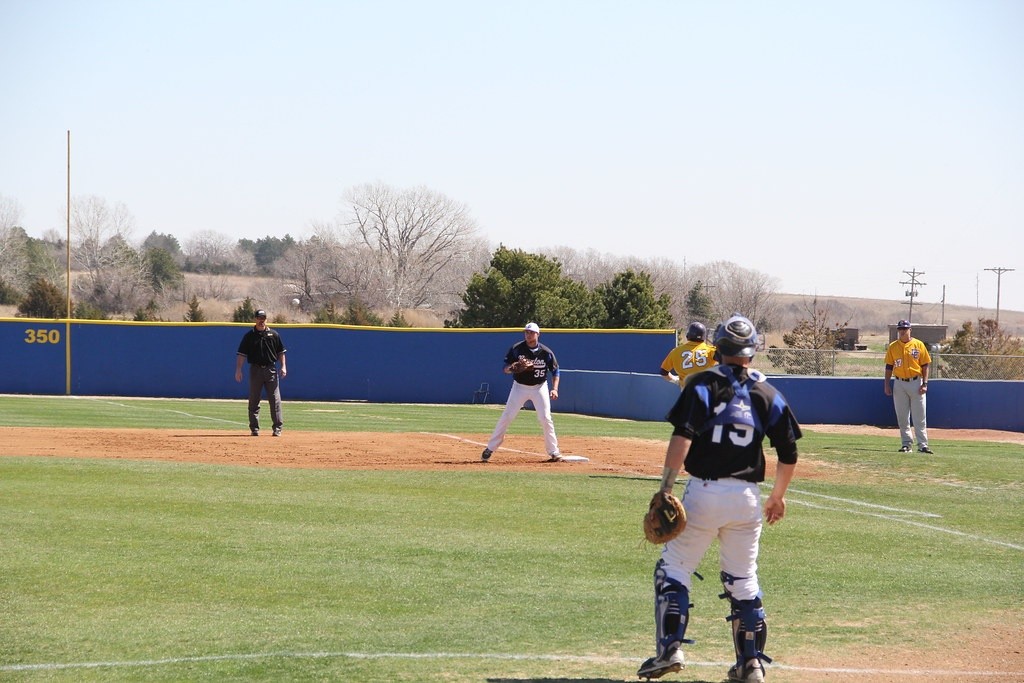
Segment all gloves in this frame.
[669,376,680,385]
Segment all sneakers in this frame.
[272,428,281,436]
[727,662,764,683]
[918,447,933,454]
[898,446,912,453]
[251,430,258,436]
[636,647,684,681]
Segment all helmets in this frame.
[685,321,706,342]
[896,320,911,329]
[712,316,757,358]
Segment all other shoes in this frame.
[551,454,564,462]
[481,448,492,461]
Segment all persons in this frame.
[660,322,719,392]
[884,320,934,454]
[637,313,803,682]
[235,309,287,436]
[482,322,563,462]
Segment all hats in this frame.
[524,322,540,333]
[255,309,267,318]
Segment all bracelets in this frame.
[667,485,673,488]
[923,382,928,385]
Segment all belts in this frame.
[895,376,917,382]
[256,363,273,369]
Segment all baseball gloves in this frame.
[643,492,687,544]
[509,358,534,374]
